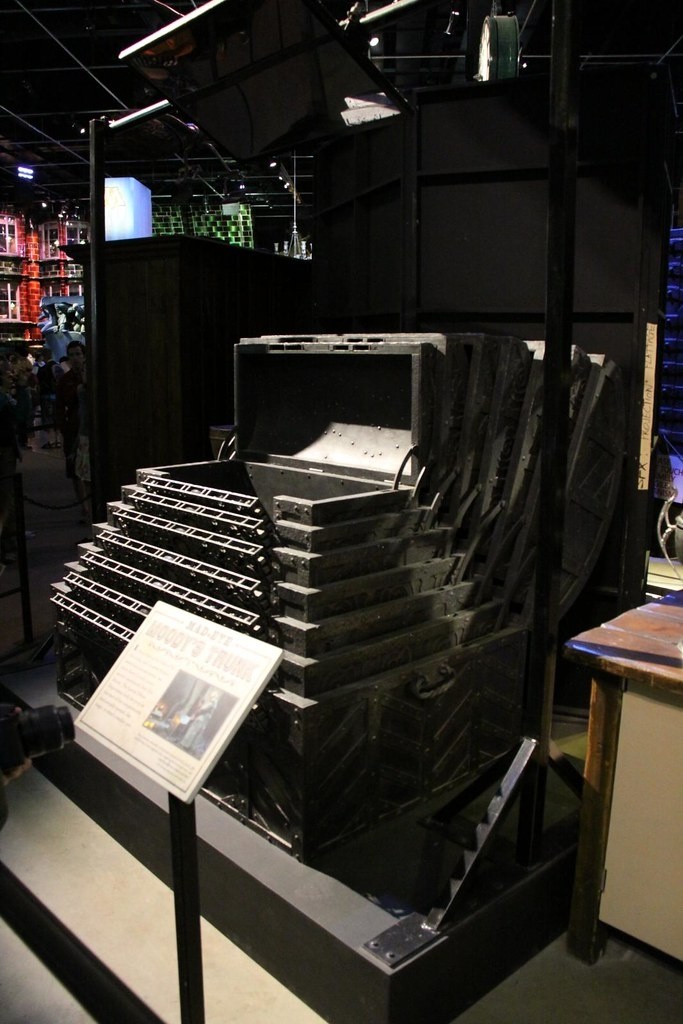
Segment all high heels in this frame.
[22,443,32,450]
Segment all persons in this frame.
[56,341,89,499]
[0,353,72,450]
[0,377,22,566]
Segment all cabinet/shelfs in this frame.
[566,596,682,965]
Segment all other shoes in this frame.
[76,515,91,526]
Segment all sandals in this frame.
[42,441,61,449]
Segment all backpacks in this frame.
[52,364,64,379]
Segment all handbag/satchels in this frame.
[69,435,90,481]
